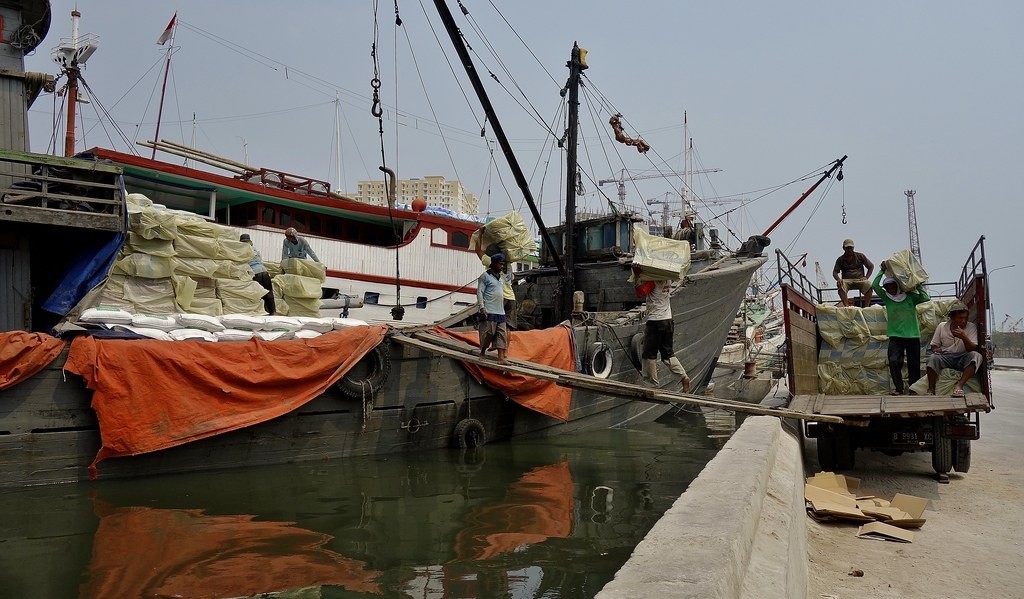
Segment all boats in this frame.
[0,0,848,488]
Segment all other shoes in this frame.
[889,388,903,395]
[907,389,917,394]
[683,377,690,392]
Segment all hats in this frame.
[881,278,900,293]
[488,252,506,267]
[240,234,249,242]
[843,239,854,248]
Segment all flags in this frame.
[155,14,176,45]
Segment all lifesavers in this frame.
[452,418,487,449]
[458,447,487,473]
[332,340,393,399]
[630,333,662,370]
[584,340,614,379]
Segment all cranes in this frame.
[650,204,724,238]
[599,167,725,213]
[647,191,751,226]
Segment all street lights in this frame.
[960,265,1016,340]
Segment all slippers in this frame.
[925,388,933,395]
[952,388,964,397]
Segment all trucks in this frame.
[774,234,995,473]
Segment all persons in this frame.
[925,305,982,396]
[681,215,694,229]
[833,239,874,307]
[239,234,276,315]
[476,253,513,366]
[282,227,320,262]
[475,227,517,359]
[631,263,690,394]
[872,260,930,394]
